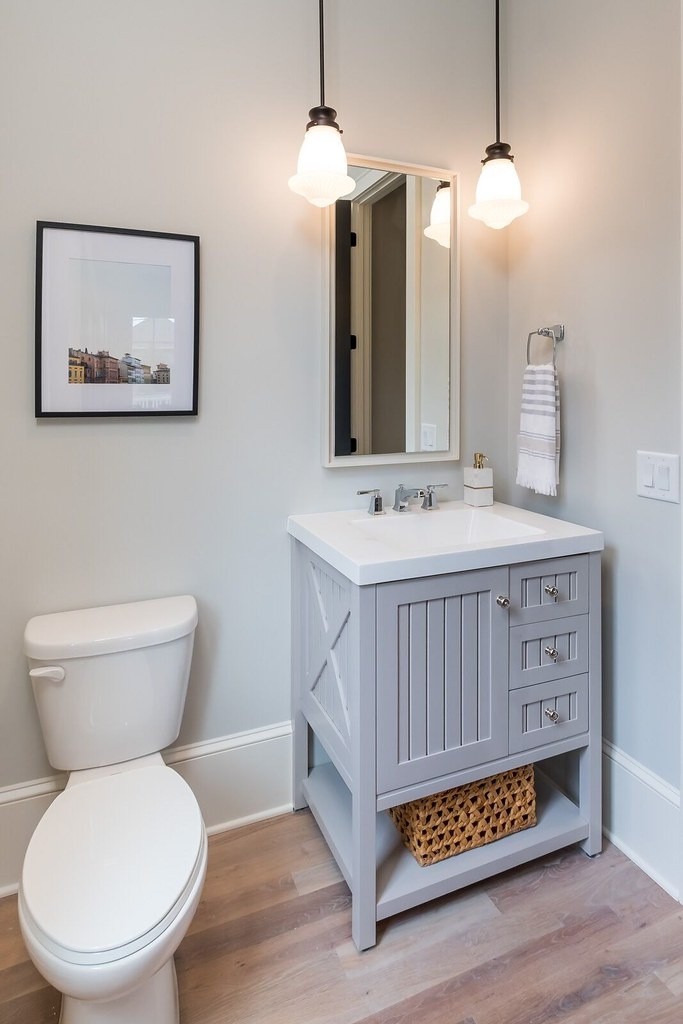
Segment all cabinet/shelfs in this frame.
[292,537,603,951]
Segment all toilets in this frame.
[16,593,210,1023]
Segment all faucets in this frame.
[391,483,430,512]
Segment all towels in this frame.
[515,361,561,497]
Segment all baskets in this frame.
[385,763,537,866]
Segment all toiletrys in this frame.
[463,452,494,508]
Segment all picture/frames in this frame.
[34,221,200,418]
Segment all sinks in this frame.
[346,507,546,557]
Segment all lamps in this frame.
[470,0,532,229]
[423,180,450,251]
[287,0,357,206]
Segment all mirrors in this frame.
[320,151,461,472]
